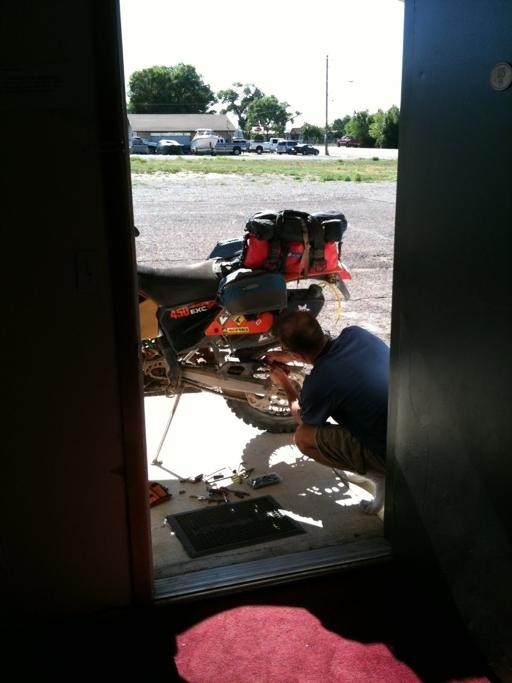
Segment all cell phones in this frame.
[247,473,281,489]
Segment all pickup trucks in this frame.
[213,138,247,155]
[249,138,284,154]
[336,135,361,147]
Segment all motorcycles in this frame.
[133,208,352,465]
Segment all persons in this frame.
[252,303,390,518]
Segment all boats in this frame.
[189,128,219,149]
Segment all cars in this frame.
[129,136,185,155]
[277,140,319,155]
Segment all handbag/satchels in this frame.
[209,211,347,315]
